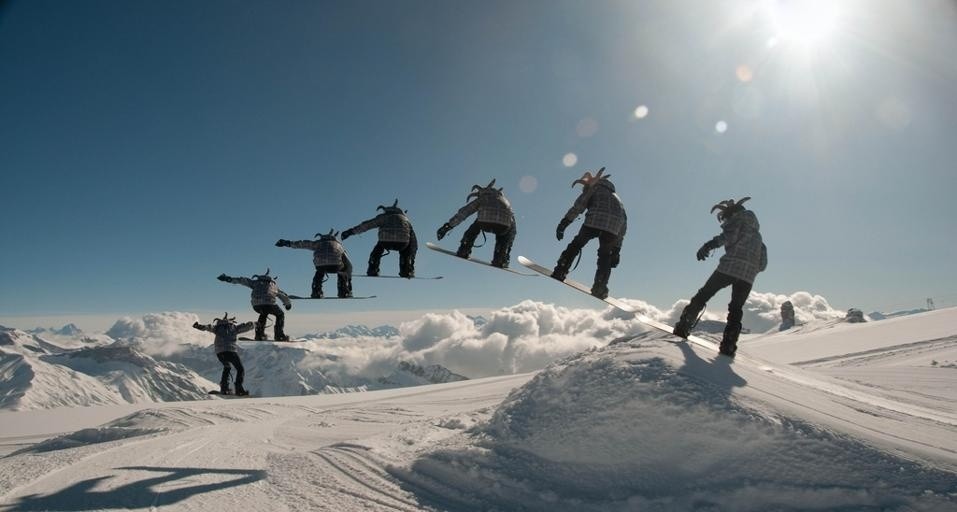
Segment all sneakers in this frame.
[491,259,507,269]
[456,250,471,259]
[236,391,249,396]
[399,271,411,279]
[274,336,289,342]
[311,292,323,298]
[719,343,737,358]
[673,323,691,339]
[338,291,352,298]
[367,269,379,276]
[255,335,267,341]
[591,286,609,299]
[221,390,232,394]
[550,267,569,282]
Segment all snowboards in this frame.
[209,390,258,398]
[239,337,308,342]
[426,242,540,276]
[518,256,638,312]
[635,312,776,371]
[288,295,378,299]
[352,275,444,279]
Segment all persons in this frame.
[341,197,419,278]
[672,196,768,356]
[550,166,628,299]
[436,177,517,268]
[274,228,353,298]
[191,311,258,395]
[216,267,292,341]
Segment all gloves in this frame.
[437,223,453,240]
[556,219,572,241]
[341,228,353,241]
[348,282,352,291]
[285,304,291,310]
[275,239,293,247]
[610,247,621,268]
[697,245,710,261]
[193,322,204,329]
[217,273,231,282]
[503,253,510,266]
